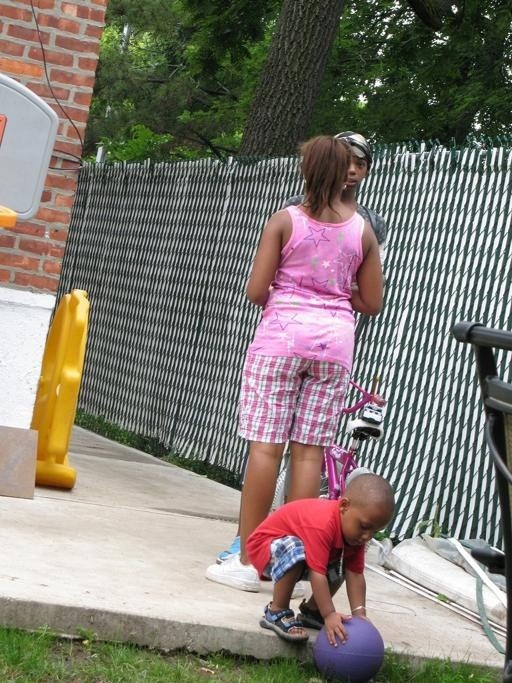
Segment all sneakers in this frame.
[205,538,260,592]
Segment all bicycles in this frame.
[265,380,386,513]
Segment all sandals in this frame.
[259,601,309,642]
[296,600,325,631]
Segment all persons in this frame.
[246,473,395,647]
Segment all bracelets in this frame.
[351,606,366,613]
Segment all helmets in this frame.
[333,131,372,169]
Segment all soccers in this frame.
[314,617,384,681]
